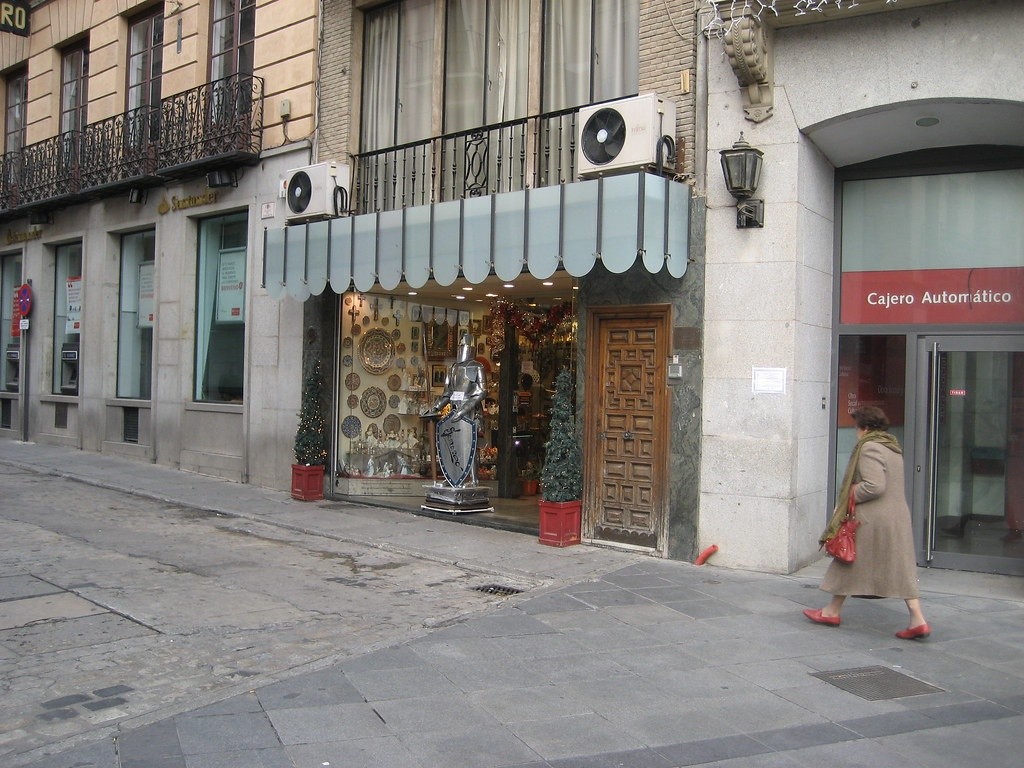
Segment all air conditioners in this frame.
[285,160,350,223]
[577,93,676,179]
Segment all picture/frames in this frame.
[432,365,447,387]
[482,314,494,335]
[469,319,482,338]
[423,306,459,361]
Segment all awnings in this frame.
[263,173,689,306]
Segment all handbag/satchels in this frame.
[824,484,862,564]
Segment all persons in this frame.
[802,406,930,640]
[366,455,413,476]
[366,426,420,449]
[422,332,488,488]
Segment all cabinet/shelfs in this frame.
[398,388,436,415]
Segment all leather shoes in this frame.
[895,624,930,639]
[803,609,841,627]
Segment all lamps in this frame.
[128,188,148,204]
[719,131,764,228]
[206,167,239,187]
[30,211,54,224]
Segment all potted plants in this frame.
[521,453,542,496]
[291,360,329,501]
[537,365,583,548]
